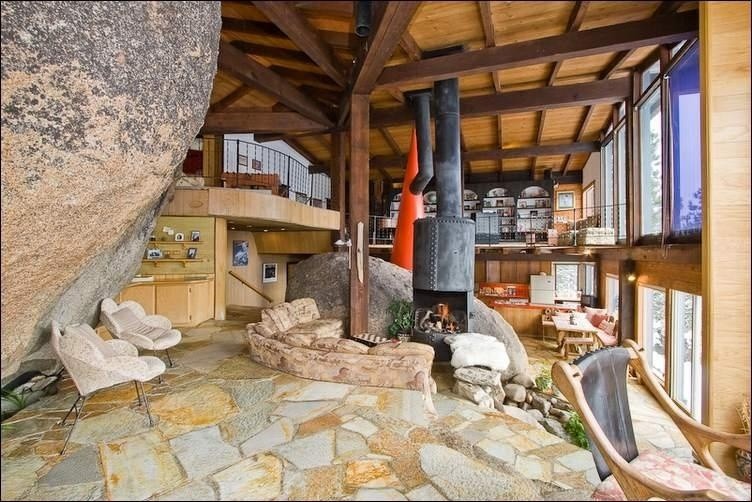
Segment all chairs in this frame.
[551,336,752,501]
[540,306,559,342]
[98,296,182,386]
[414,306,469,361]
[44,318,168,454]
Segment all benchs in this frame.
[592,323,618,349]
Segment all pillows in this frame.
[584,307,616,336]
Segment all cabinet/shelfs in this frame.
[121,277,219,328]
[386,180,553,243]
[475,281,530,305]
[142,240,208,267]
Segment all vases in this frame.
[386,297,416,344]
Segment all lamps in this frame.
[354,1,374,39]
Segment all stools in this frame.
[577,227,617,247]
[555,230,581,245]
[561,336,595,361]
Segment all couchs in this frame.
[258,295,346,342]
[243,320,439,394]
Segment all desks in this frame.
[551,314,598,348]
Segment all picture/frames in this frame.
[174,231,186,243]
[286,259,298,286]
[188,247,198,260]
[261,262,278,284]
[237,154,249,167]
[251,159,262,171]
[145,248,164,261]
[555,190,575,212]
[189,230,201,242]
